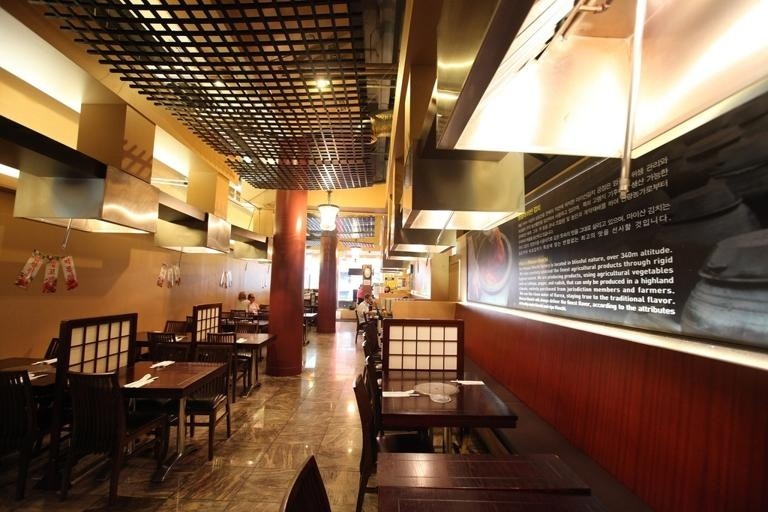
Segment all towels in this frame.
[122,375,156,390]
[151,360,175,369]
[382,390,415,398]
[454,380,484,386]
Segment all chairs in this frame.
[231,309,246,320]
[61,370,170,506]
[365,353,428,436]
[43,338,60,360]
[259,305,269,308]
[164,321,186,333]
[368,321,382,351]
[186,316,193,331]
[363,332,382,378]
[130,342,192,459]
[278,455,332,512]
[146,332,175,361]
[362,340,382,396]
[254,316,262,320]
[221,312,232,320]
[364,324,382,364]
[0,369,73,500]
[207,332,249,403]
[235,323,259,386]
[246,313,254,319]
[355,309,366,344]
[352,373,435,512]
[221,317,228,332]
[160,344,234,461]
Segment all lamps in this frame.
[317,191,340,231]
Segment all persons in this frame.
[357,294,374,329]
[248,293,260,316]
[384,286,391,294]
[234,291,250,314]
